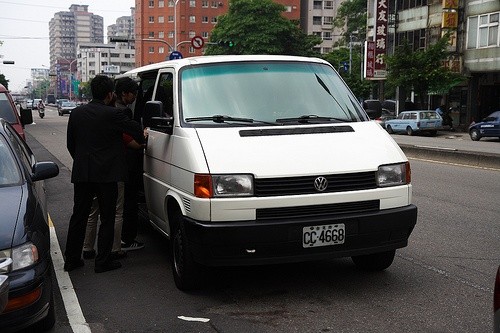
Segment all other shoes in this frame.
[110,250,129,260]
[64,258,85,271]
[95,258,122,273]
[82,248,96,260]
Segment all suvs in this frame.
[384,110,443,136]
[360,99,414,120]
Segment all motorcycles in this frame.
[38,105,45,119]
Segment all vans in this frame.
[0,82,33,143]
[112,51,419,292]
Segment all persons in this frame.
[37,101,46,112]
[436,106,442,115]
[63,75,149,272]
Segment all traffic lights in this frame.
[217,39,237,47]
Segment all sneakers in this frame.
[120,240,145,250]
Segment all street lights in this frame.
[349,30,359,80]
[53,54,87,101]
[42,64,67,103]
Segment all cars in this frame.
[468,110,500,142]
[0,117,60,333]
[14,94,88,116]
